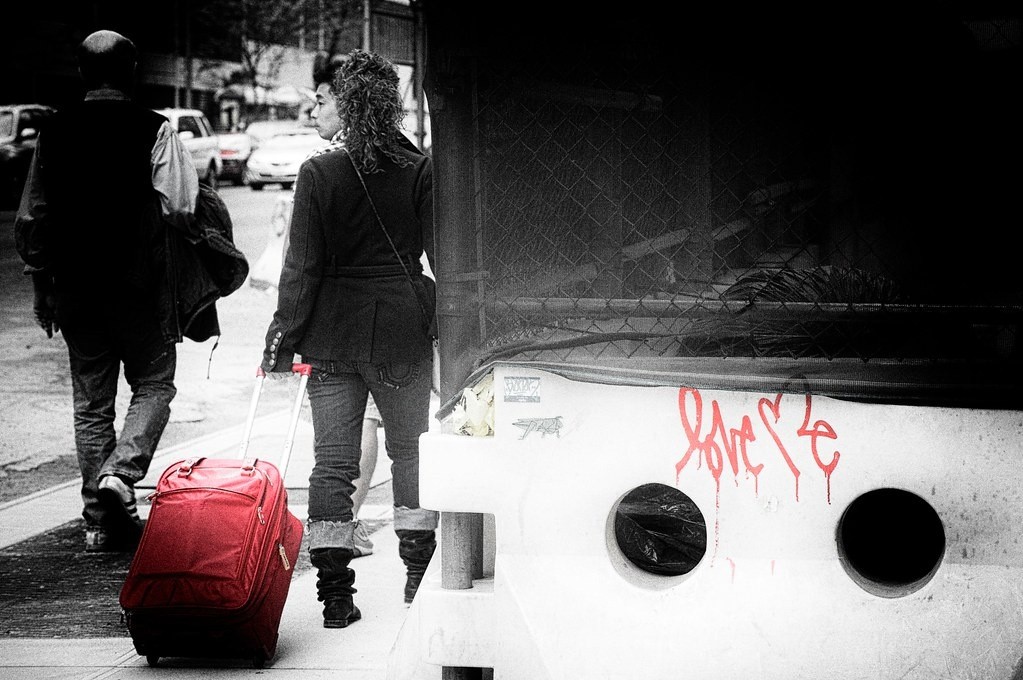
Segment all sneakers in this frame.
[96,477,142,537]
[83,529,106,551]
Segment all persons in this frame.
[258,51,437,629]
[13,28,199,552]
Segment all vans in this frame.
[152,108,224,189]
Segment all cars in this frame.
[207,119,252,185]
[0,104,59,206]
[243,121,333,189]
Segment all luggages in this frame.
[117,360,314,668]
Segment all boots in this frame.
[309,548,360,628]
[396,527,437,603]
